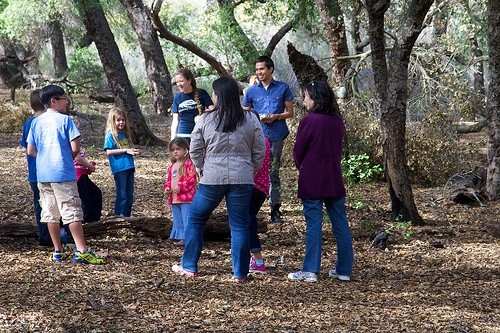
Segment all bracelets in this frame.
[278,114,281,120]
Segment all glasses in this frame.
[55,96,69,100]
[308,79,315,85]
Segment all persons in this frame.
[246,110,271,274]
[20,89,64,250]
[242,55,293,225]
[26,85,105,265]
[171,77,266,283]
[164,136,197,246]
[103,107,139,218]
[288,80,353,282]
[169,68,215,187]
[72,150,102,224]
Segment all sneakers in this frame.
[288,271,318,282]
[329,270,350,281]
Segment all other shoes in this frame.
[172,264,198,277]
[73,248,106,265]
[249,258,266,274]
[270,203,282,222]
[51,249,71,262]
[232,276,246,283]
[174,240,184,245]
[37,245,48,250]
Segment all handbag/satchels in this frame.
[193,92,210,126]
